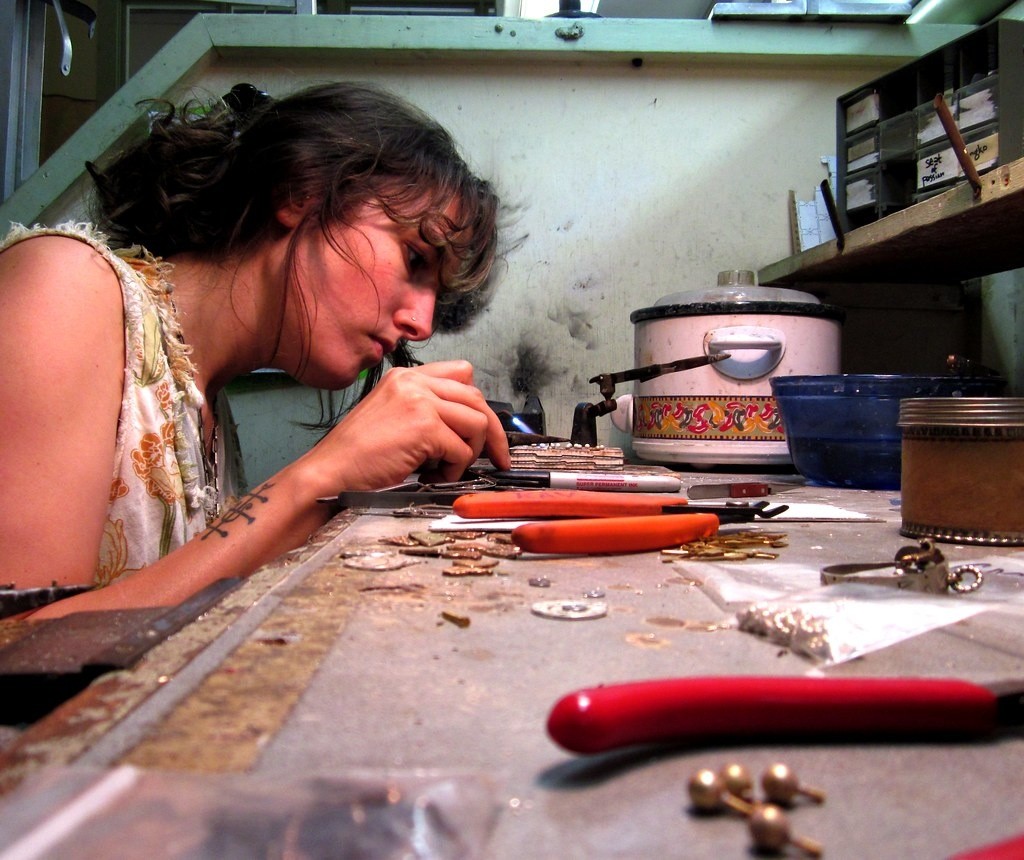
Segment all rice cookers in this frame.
[610,270,842,471]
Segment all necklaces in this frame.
[172,290,223,526]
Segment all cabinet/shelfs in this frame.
[833,20,1024,240]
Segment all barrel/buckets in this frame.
[896,397,1024,544]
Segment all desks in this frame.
[0,470,1024,860]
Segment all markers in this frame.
[475,470,682,492]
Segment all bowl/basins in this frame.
[770,373,1004,489]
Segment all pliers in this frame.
[452,491,790,557]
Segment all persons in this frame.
[0,82,511,624]
